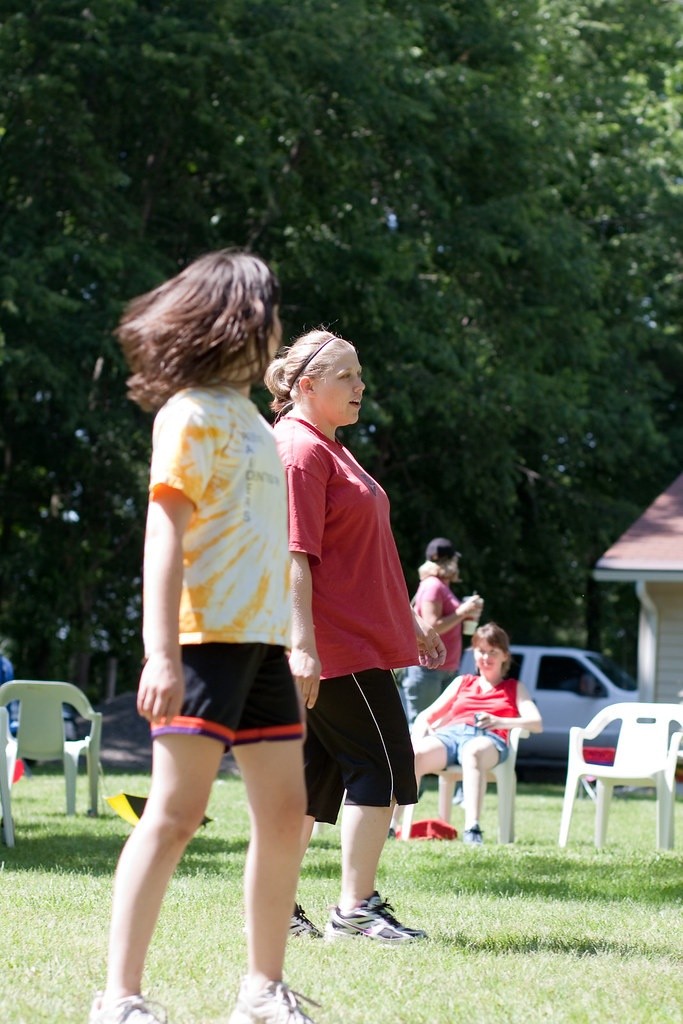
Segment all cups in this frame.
[463,597,483,634]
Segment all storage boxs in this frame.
[584,745,616,767]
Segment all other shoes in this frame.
[466,825,483,844]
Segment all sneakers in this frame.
[289,905,324,937]
[90,995,168,1024]
[226,985,319,1024]
[324,891,426,944]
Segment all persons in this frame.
[261,331,446,946]
[404,538,480,721]
[388,623,543,847]
[89,255,318,1024]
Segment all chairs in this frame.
[0,680,102,847]
[559,703,682,851]
[400,726,529,844]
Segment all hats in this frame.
[426,537,455,562]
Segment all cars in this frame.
[448,646,641,784]
